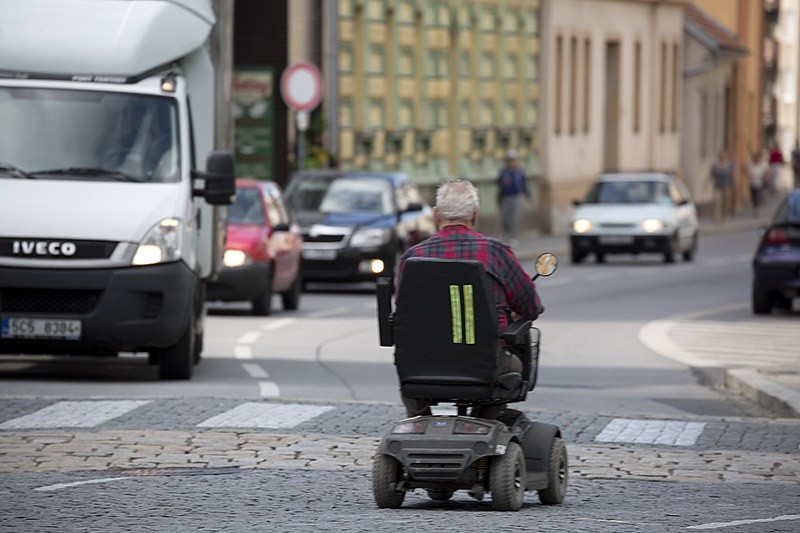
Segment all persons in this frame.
[496,149,534,245]
[305,134,332,171]
[393,178,546,420]
[702,140,784,221]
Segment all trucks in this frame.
[0,0,245,380]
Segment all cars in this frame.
[749,189,800,315]
[282,169,435,290]
[567,170,702,266]
[205,179,304,315]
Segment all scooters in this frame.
[371,252,570,510]
